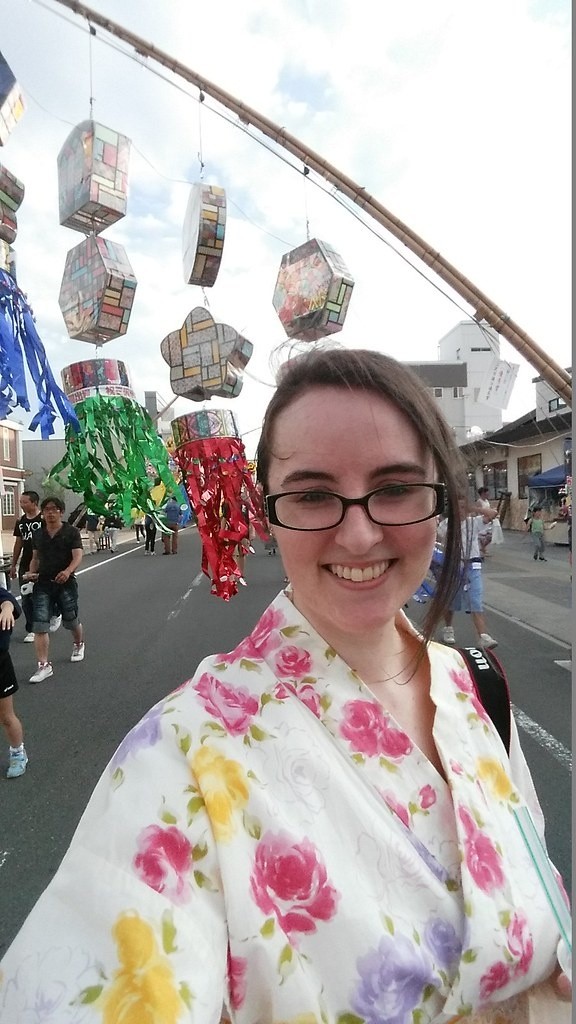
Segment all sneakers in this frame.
[7,749,28,777]
[442,626,455,643]
[23,632,34,642]
[29,661,53,682]
[475,636,497,651]
[71,640,85,662]
[49,614,62,631]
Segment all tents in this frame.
[527,462,572,507]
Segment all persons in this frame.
[86,508,118,554]
[436,494,498,650]
[525,506,554,561]
[163,497,182,555]
[475,486,493,557]
[29,496,85,683]
[218,488,276,581]
[0,349,572,1024]
[134,508,145,543]
[0,588,29,778]
[144,513,157,556]
[8,490,61,641]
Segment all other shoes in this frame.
[151,551,155,555]
[136,540,140,544]
[163,553,169,555]
[534,553,537,560]
[539,557,546,561]
[173,551,177,554]
[145,551,149,555]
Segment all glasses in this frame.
[264,482,448,531]
